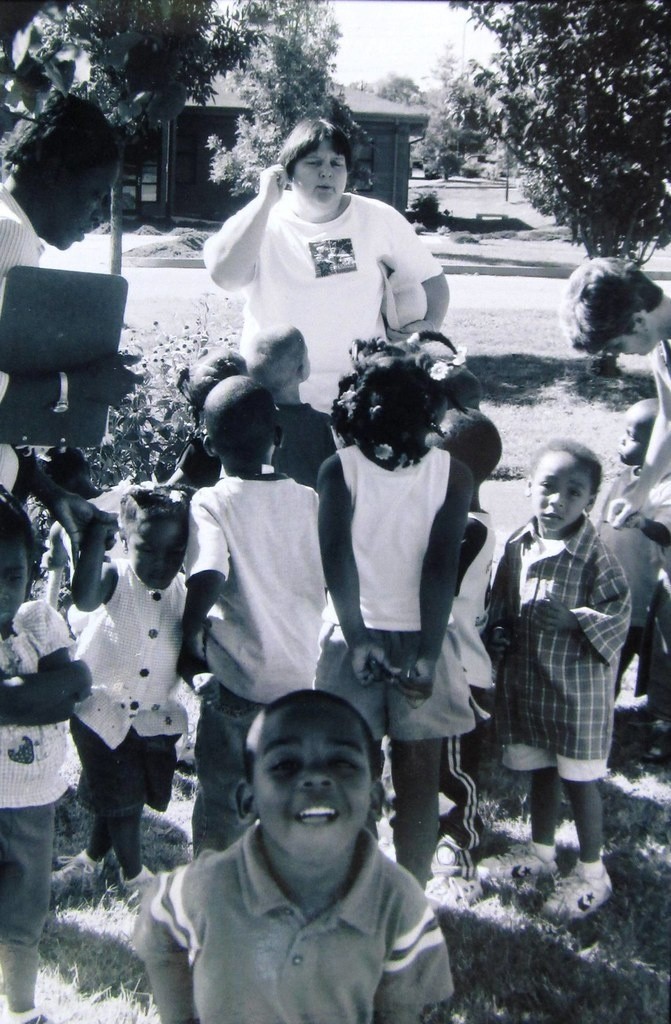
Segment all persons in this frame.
[133,690,454,1024]
[0,91,671,1024]
[204,118,450,415]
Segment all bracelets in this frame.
[426,319,438,335]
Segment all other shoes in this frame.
[640,726,671,764]
[24,1014,54,1024]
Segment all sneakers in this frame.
[51,856,104,887]
[540,864,613,919]
[424,872,484,912]
[119,865,156,896]
[477,844,560,880]
